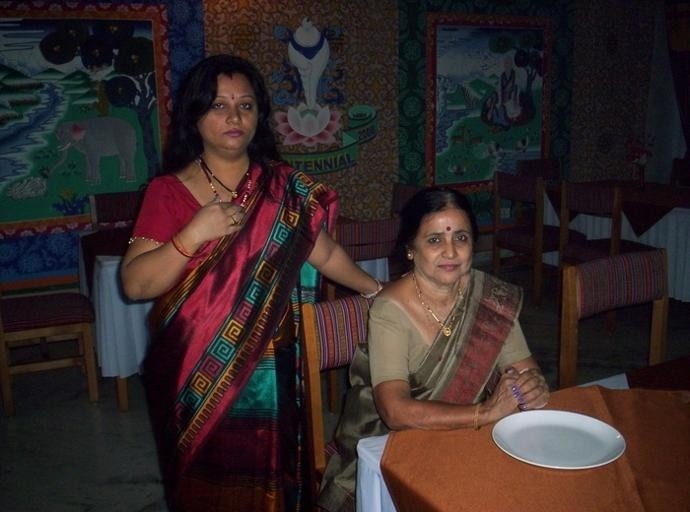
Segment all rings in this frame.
[229,214,237,224]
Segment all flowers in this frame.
[619,131,658,180]
[53,186,90,215]
[275,100,341,150]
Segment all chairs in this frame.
[91,190,143,229]
[558,181,656,263]
[0,293,99,417]
[558,248,666,390]
[376,226,679,297]
[527,155,559,182]
[323,219,402,302]
[303,294,374,511]
[492,173,585,306]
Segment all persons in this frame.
[309,182,553,511]
[115,53,384,512]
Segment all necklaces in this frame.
[407,268,465,338]
[195,154,239,202]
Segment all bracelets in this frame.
[472,401,482,432]
[359,277,383,299]
[170,234,196,258]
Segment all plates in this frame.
[491,409,626,470]
